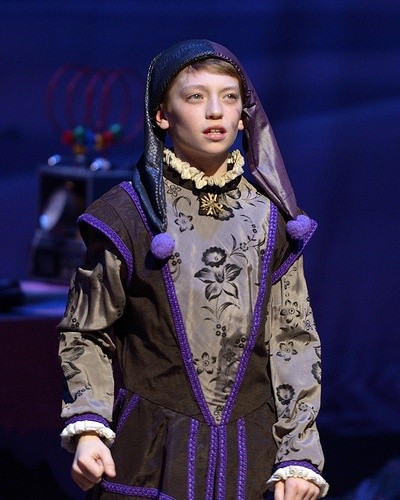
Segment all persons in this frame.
[54,40,330,500]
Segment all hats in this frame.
[134,38,314,259]
